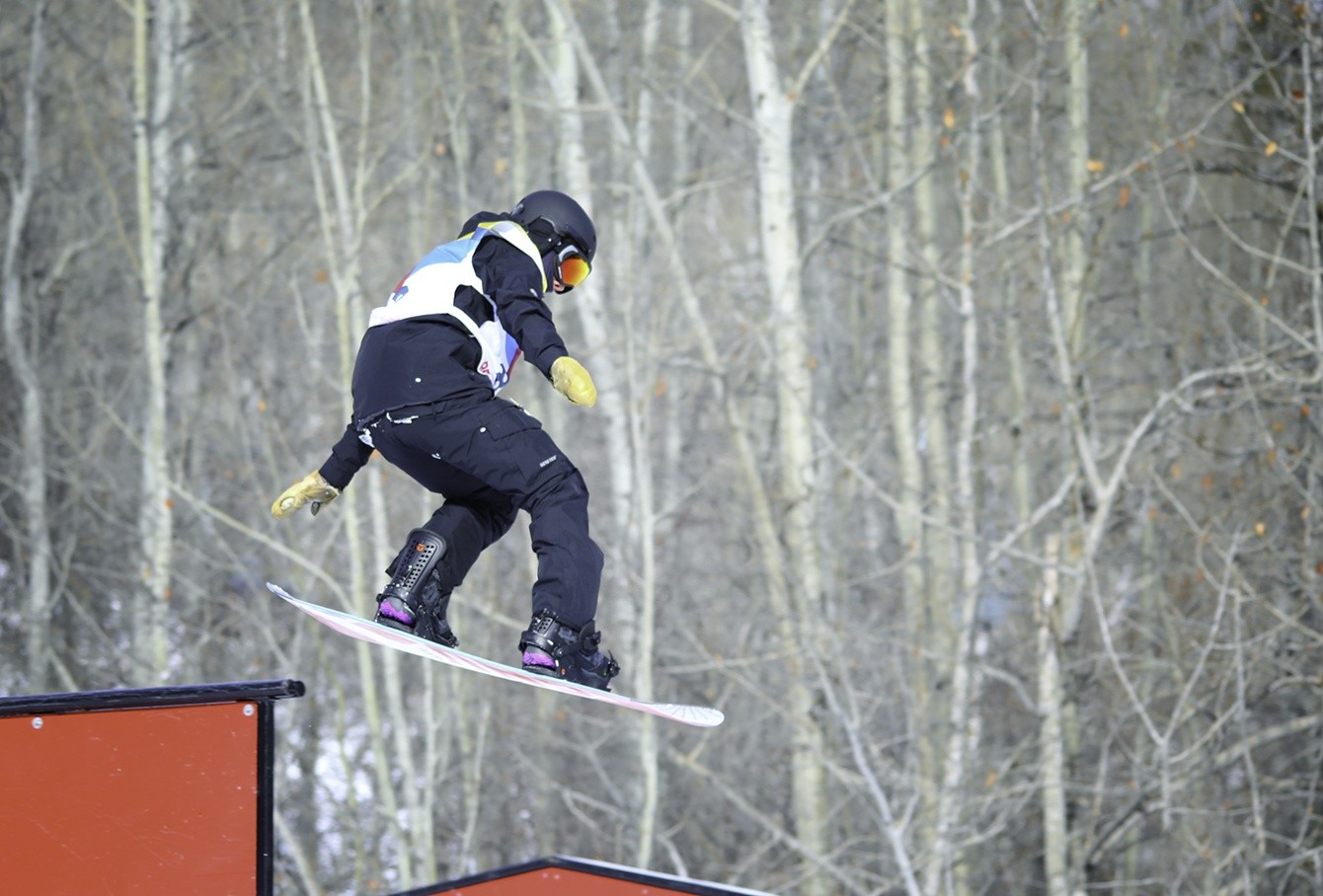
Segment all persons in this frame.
[271,191,604,678]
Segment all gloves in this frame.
[549,355,597,407]
[270,470,341,518]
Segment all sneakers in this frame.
[376,528,460,649]
[517,606,622,693]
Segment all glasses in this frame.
[554,237,592,295]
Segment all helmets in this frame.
[513,190,597,294]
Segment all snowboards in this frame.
[266,580,727,730]
